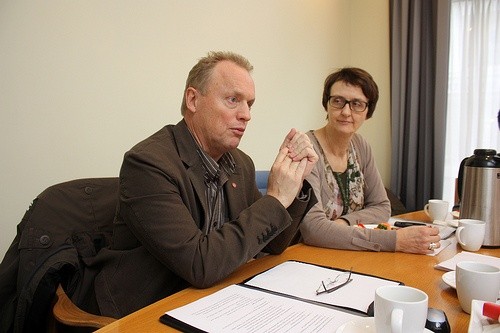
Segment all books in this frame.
[159,260,403,333]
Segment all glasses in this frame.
[316,265,353,295]
[328,95,369,111]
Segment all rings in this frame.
[429,243,436,249]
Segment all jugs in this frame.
[457,150,500,249]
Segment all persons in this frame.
[298,68,441,254]
[79,52,319,333]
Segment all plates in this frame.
[442,271,456,289]
[468,300,500,333]
[335,317,434,333]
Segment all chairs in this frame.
[1,177,120,333]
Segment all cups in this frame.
[456,262,500,315]
[456,219,486,252]
[424,200,449,222]
[375,286,429,333]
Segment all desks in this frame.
[93,210,500,333]
